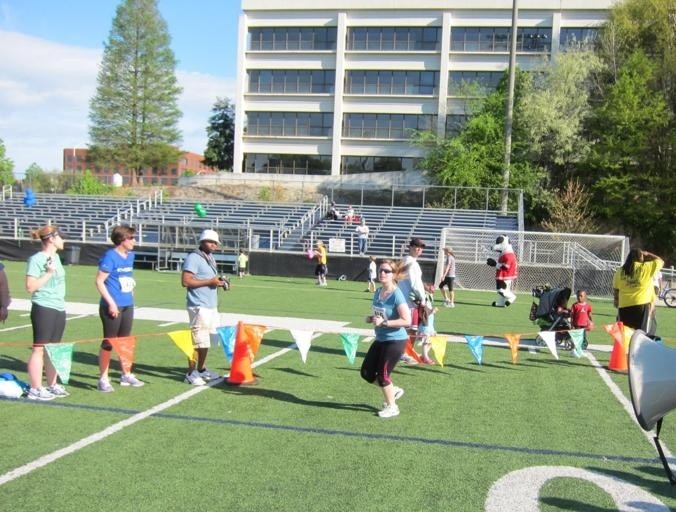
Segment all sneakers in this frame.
[377,405,400,417]
[184,369,206,386]
[27,385,57,402]
[120,372,143,388]
[96,379,114,392]
[445,301,455,308]
[420,355,435,365]
[382,386,404,406]
[46,384,70,398]
[198,367,220,380]
[441,298,450,305]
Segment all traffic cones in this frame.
[225,321,261,389]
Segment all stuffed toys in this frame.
[487,234,517,307]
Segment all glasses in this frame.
[122,235,136,241]
[378,268,393,273]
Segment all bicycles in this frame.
[658,276,676,308]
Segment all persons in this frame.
[612,247,665,334]
[181,229,231,386]
[313,240,328,286]
[95,225,145,392]
[364,256,377,292]
[396,237,437,365]
[356,218,368,256]
[237,248,248,278]
[25,224,71,401]
[571,289,592,349]
[327,205,353,221]
[360,259,412,418]
[439,246,455,308]
[0,262,11,326]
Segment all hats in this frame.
[442,246,456,253]
[198,229,222,248]
[409,237,426,248]
[41,226,71,240]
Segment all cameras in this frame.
[49,256,55,264]
[218,276,230,290]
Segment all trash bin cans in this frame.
[64,246,81,264]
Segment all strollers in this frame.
[529,285,589,352]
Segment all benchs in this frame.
[1,186,516,266]
